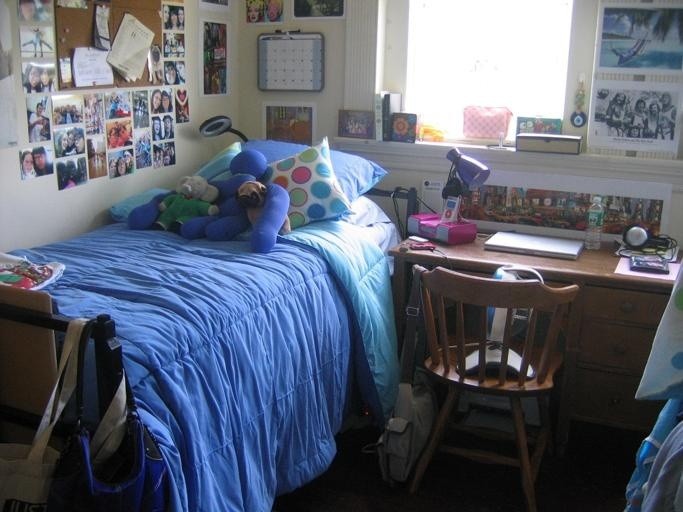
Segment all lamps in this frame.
[197,115,247,142]
[406,149,490,241]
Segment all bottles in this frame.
[583,194,605,251]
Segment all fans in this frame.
[455,264,548,381]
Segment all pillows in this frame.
[239,132,389,226]
[194,140,241,184]
[105,186,172,223]
[240,136,357,232]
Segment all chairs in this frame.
[409,261,579,511]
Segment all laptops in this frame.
[484,231,585,261]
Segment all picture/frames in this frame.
[197,16,232,98]
[261,101,317,149]
[290,1,346,22]
[198,0,231,16]
[243,0,283,27]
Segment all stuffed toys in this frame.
[153,175,220,232]
[235,180,291,235]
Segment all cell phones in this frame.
[410,242,435,250]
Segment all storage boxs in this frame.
[512,131,583,155]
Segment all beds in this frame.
[1,186,417,512]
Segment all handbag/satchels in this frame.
[363,365,440,494]
[0,316,167,511]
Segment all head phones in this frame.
[622,223,670,249]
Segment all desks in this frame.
[383,225,682,462]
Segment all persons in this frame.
[605,91,676,140]
[17,1,189,189]
